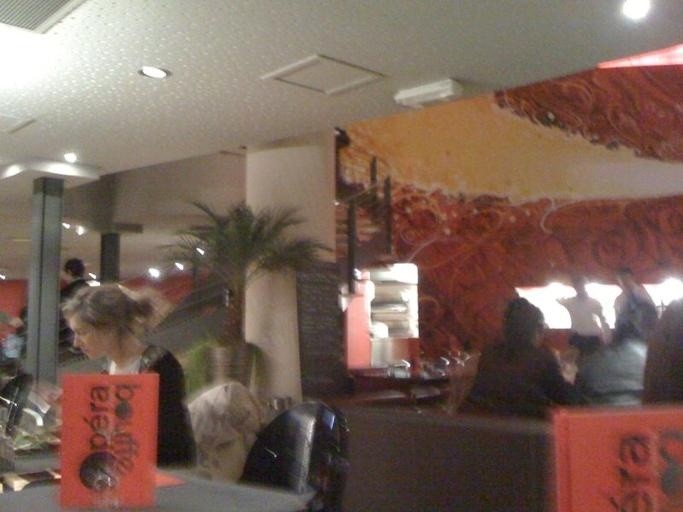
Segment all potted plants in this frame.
[155,191,334,384]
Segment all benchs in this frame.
[329,400,559,511]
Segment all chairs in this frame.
[185,383,265,483]
[0,371,32,438]
[233,400,350,511]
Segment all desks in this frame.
[0,454,308,512]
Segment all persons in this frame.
[643,293,681,408]
[0,310,25,328]
[610,268,657,322]
[458,295,587,420]
[573,299,662,405]
[59,283,198,471]
[57,256,87,349]
[555,275,611,350]
